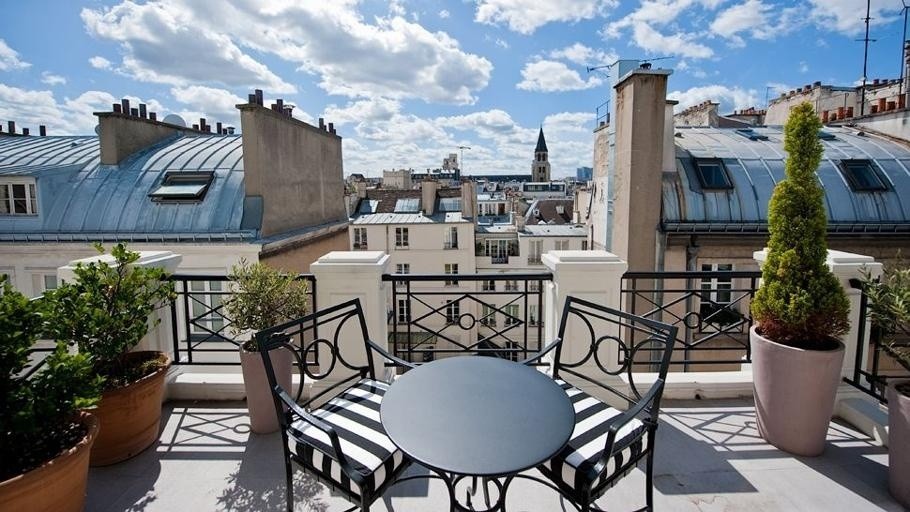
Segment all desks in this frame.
[345,354,582,512]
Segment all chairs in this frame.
[254,296,418,511]
[520,294,679,512]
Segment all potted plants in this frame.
[857,247,910,510]
[748,99,851,458]
